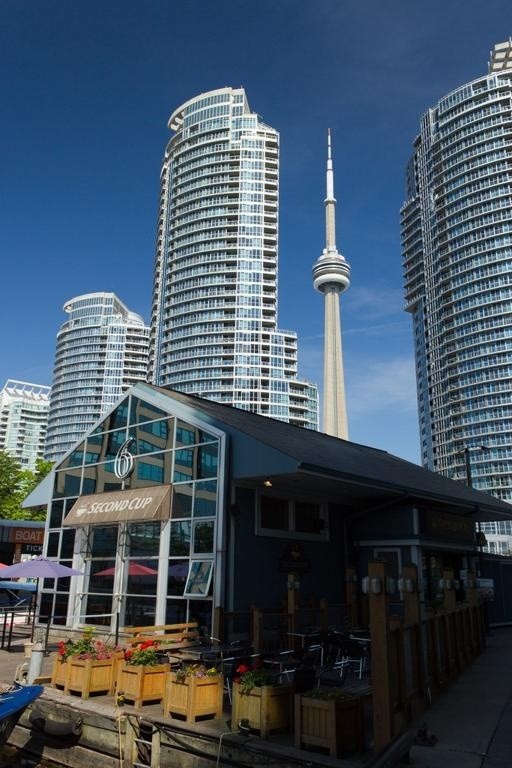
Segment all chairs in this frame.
[151,625,371,712]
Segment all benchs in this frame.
[123,622,200,668]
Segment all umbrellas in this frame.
[91,561,159,577]
[167,562,191,576]
[0,554,86,642]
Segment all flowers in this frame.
[56,624,160,666]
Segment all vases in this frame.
[48,652,122,703]
[115,659,170,710]
[292,691,366,759]
[163,667,224,725]
[230,680,293,738]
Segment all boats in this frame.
[0,685,44,750]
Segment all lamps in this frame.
[361,576,460,596]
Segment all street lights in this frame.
[454,445,491,489]
